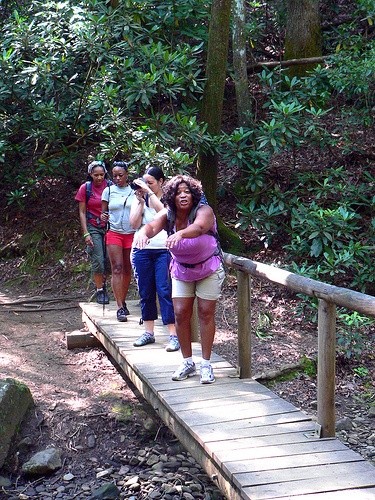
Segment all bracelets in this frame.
[148,192,155,197]
[83,233,90,238]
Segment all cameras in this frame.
[130,178,147,190]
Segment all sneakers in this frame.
[96,288,110,304]
[199,365,215,384]
[171,361,197,380]
[117,307,127,322]
[133,332,155,346]
[165,335,180,351]
[122,302,129,314]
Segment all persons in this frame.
[130,166,181,352]
[101,161,137,322]
[143,175,225,384]
[75,161,114,304]
